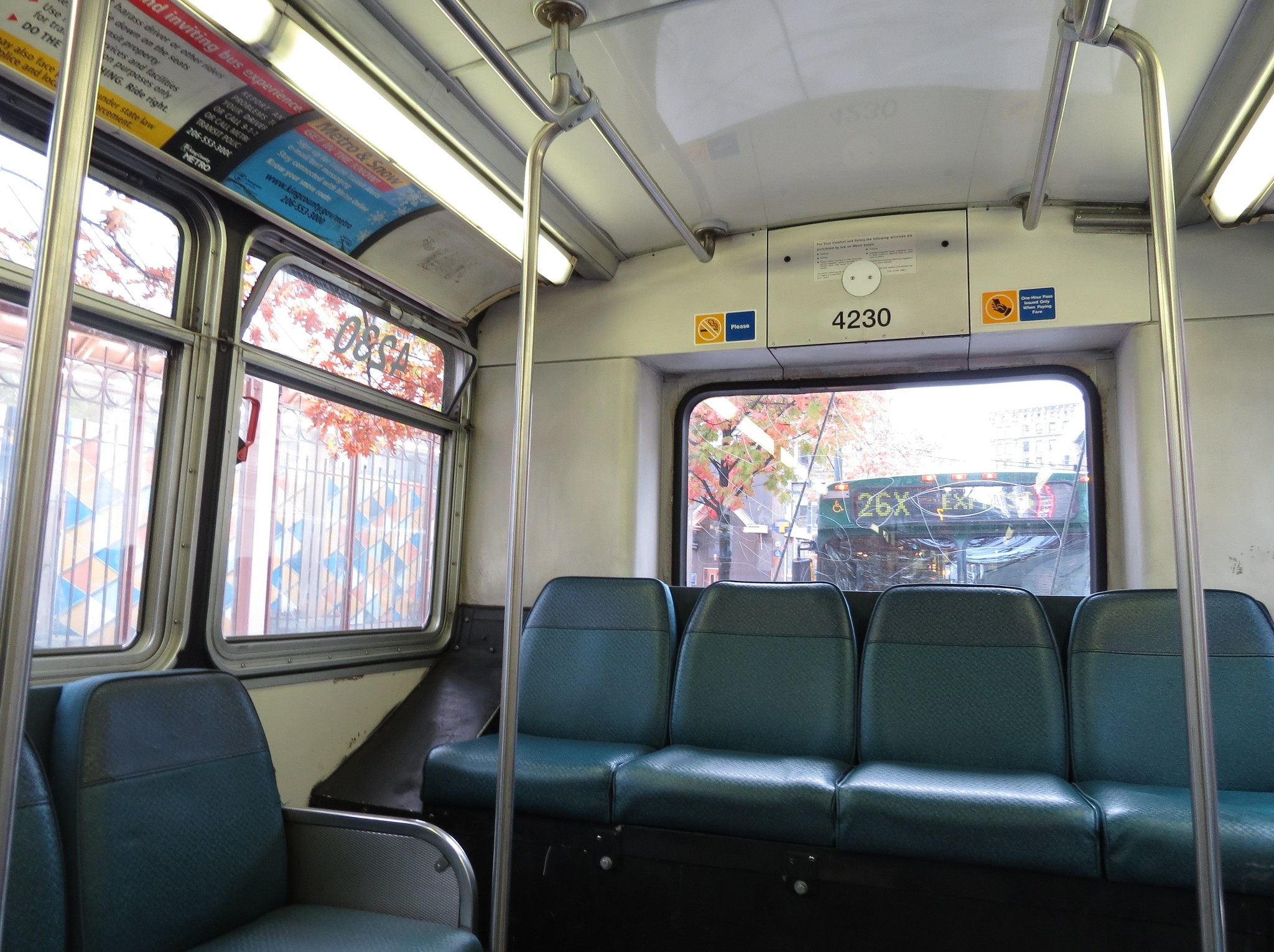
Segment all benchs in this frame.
[418,575,1274,952]
[1,669,483,952]
